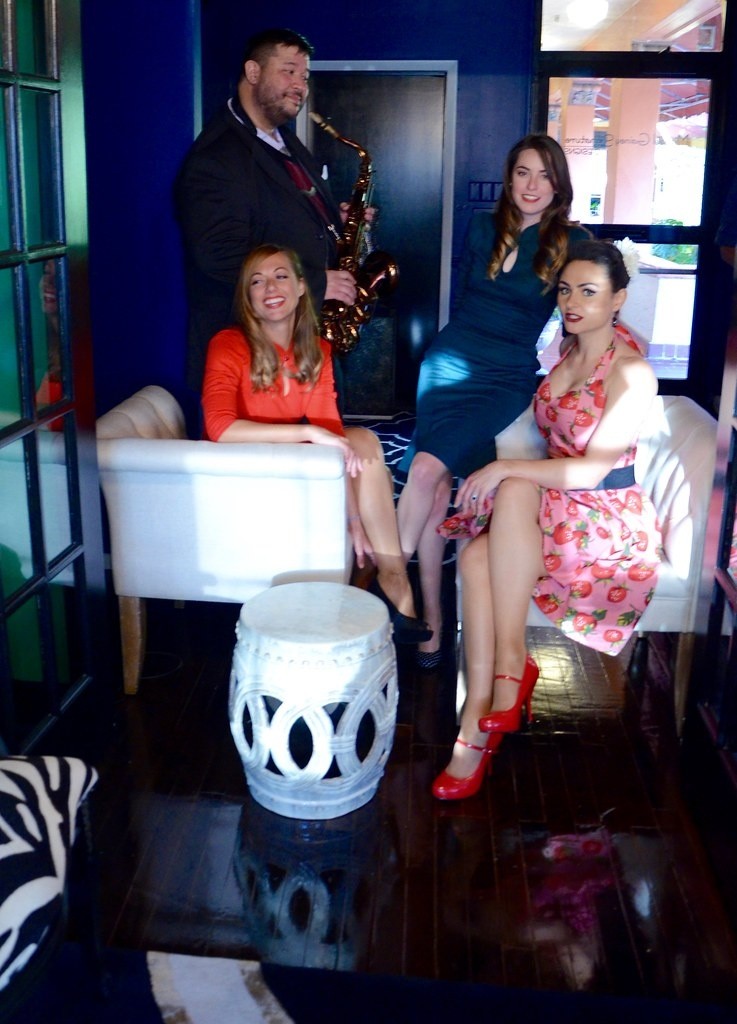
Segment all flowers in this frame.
[613,236,641,278]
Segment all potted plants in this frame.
[535,306,564,374]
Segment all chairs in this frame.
[456,395,720,737]
[94,386,356,693]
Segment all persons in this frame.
[396,136,592,669]
[432,243,662,798]
[202,245,435,646]
[534,830,613,986]
[175,28,375,394]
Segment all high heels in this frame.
[478,652,539,733]
[433,732,504,801]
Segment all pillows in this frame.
[0,754,98,1024]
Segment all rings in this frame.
[472,495,477,500]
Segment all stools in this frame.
[230,583,399,821]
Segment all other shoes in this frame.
[368,574,434,643]
[416,621,444,669]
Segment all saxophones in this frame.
[306,110,402,353]
